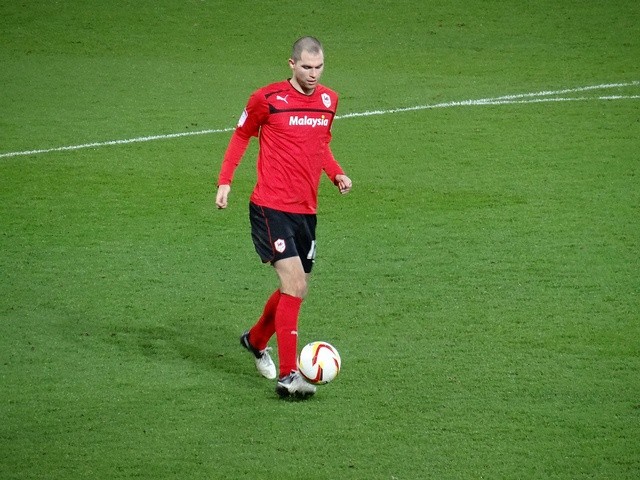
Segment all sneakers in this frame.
[238,332,277,380]
[275,372,316,398]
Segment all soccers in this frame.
[296,341,341,386]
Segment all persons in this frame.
[215,36,353,399]
